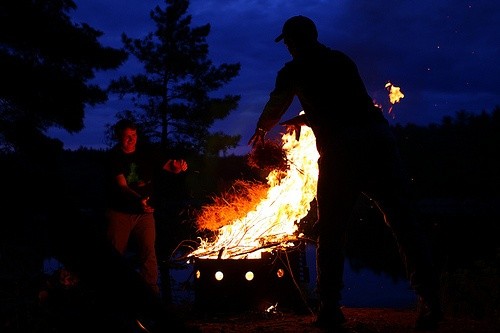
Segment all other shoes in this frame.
[308,303,345,325]
[416,305,444,332]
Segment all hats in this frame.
[274,14,317,42]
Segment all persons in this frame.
[100,119,188,303]
[248,14,454,333]
